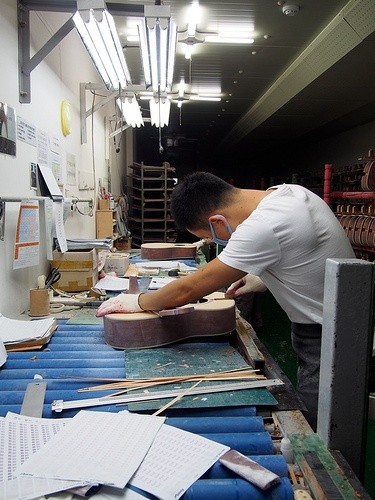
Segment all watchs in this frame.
[201,240,206,246]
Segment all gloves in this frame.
[96,292,145,317]
[225,272,267,298]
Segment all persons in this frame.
[95,170,357,433]
[194,239,254,323]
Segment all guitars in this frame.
[141,242,197,261]
[360,160,375,192]
[332,211,375,249]
[102,290,237,351]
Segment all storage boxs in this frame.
[50,248,99,291]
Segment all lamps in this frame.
[70,0,177,128]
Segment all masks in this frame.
[210,222,234,246]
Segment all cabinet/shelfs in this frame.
[96,209,118,250]
